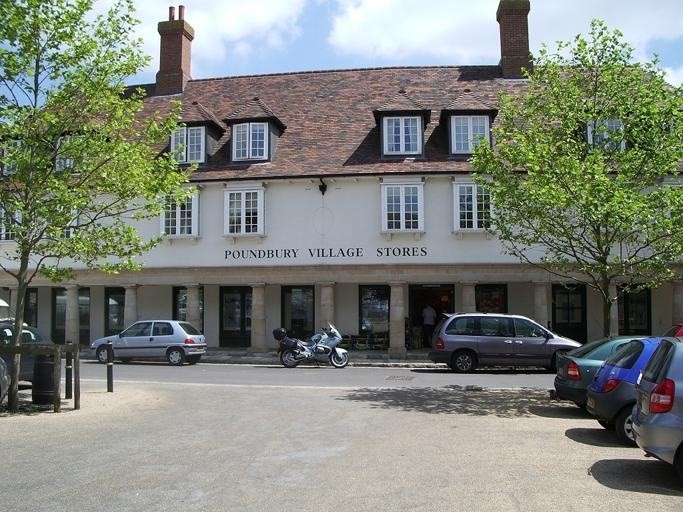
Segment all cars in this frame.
[630,338,682,483]
[0,322,59,388]
[660,323,682,337]
[87,320,207,366]
[584,337,667,448]
[548,336,645,409]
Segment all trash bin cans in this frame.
[32,354,61,405]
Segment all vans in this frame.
[426,309,580,375]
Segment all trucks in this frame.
[54,295,118,328]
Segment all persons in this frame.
[423,302,437,347]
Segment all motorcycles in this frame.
[271,321,349,369]
